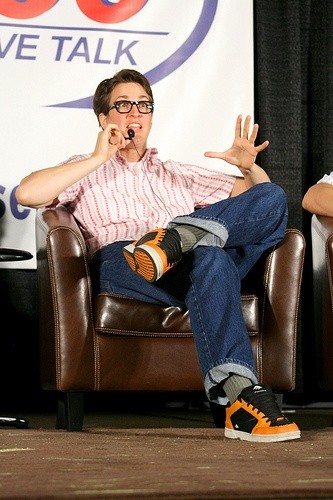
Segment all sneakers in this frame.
[224,384,301,443]
[122,227,183,284]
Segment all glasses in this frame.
[108,100,155,114]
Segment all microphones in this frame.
[128,129,135,138]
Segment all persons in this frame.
[301,171,333,219]
[15,69,300,444]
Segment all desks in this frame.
[0,247,33,430]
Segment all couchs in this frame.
[300,213,333,402]
[35,199,306,434]
